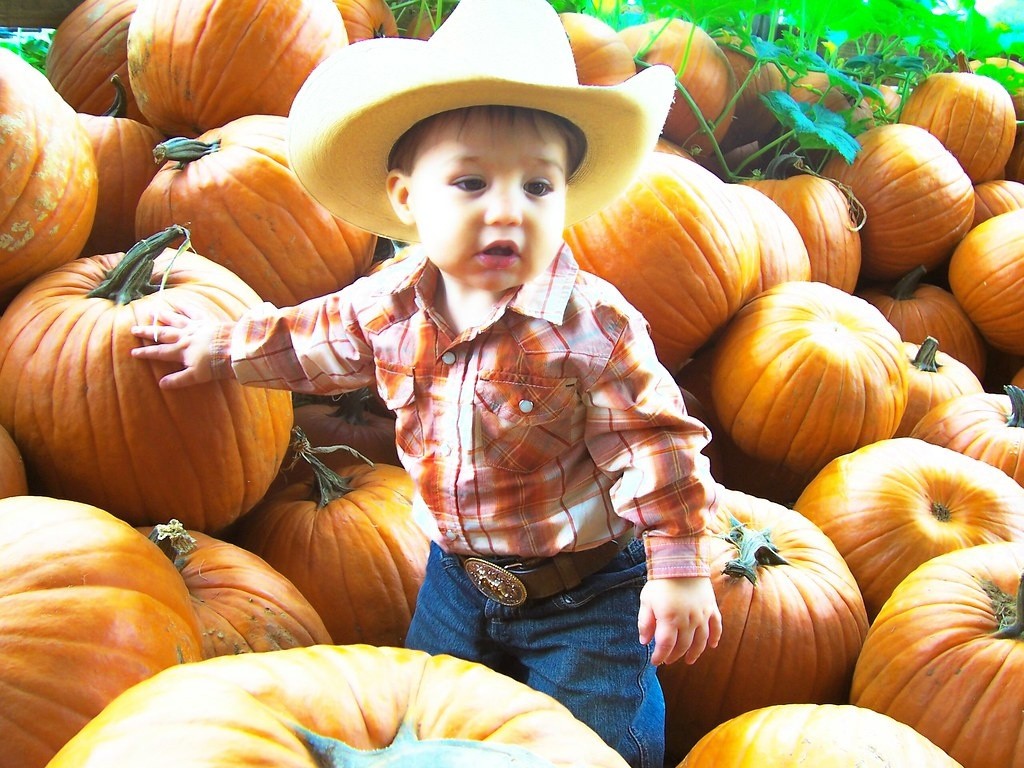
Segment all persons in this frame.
[130,0,724,768]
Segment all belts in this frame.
[454,525,635,607]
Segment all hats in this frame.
[282,0,676,245]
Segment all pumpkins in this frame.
[0,0,1024,768]
